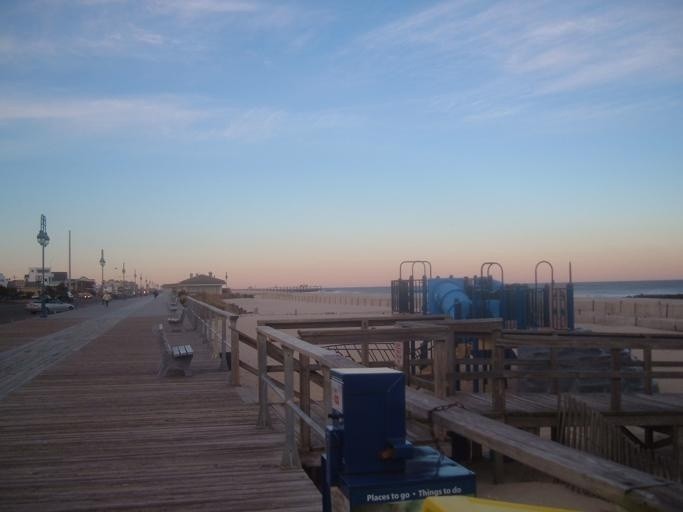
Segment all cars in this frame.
[24,297,74,315]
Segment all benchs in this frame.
[151,322,195,378]
[166,290,191,333]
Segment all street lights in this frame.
[36,214,49,319]
[98,247,105,306]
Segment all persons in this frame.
[102,291,112,307]
